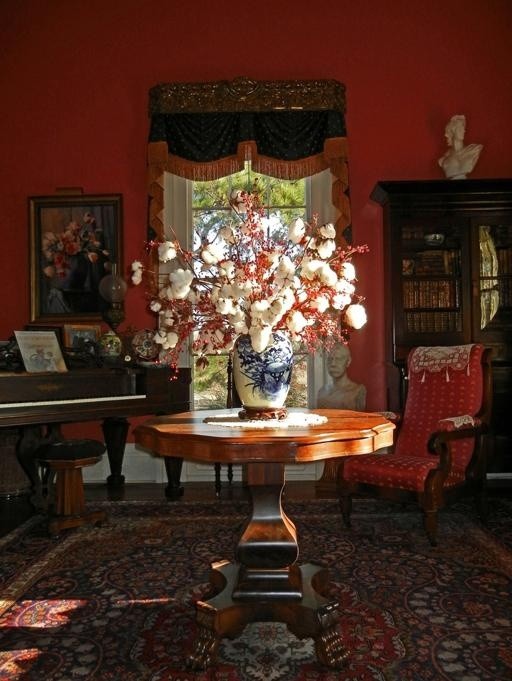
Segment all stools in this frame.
[35,436,107,540]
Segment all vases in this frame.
[232,324,294,411]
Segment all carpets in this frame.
[1,496,510,681]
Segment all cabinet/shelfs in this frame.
[369,179,512,475]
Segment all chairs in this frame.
[335,341,494,547]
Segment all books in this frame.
[14,330,69,374]
[402,224,511,335]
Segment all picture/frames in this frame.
[62,323,102,357]
[25,192,124,325]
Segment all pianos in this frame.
[1,324,192,501]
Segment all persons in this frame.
[315,341,374,411]
[436,114,483,178]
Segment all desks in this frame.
[132,407,397,672]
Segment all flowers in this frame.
[130,186,371,382]
